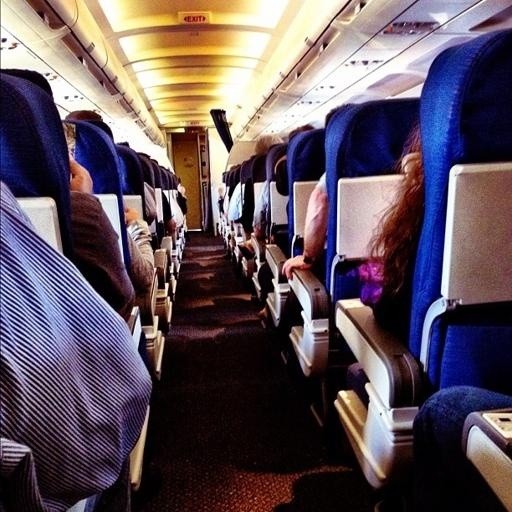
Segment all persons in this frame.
[143,184,187,234]
[69,157,136,322]
[66,110,155,290]
[229,124,316,241]
[344,124,426,380]
[0,182,151,512]
[280,171,329,283]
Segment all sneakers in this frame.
[238,242,254,261]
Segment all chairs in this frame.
[215,30,325,332]
[286,97,426,435]
[2,67,187,510]
[334,28,509,510]
[407,384,510,510]
